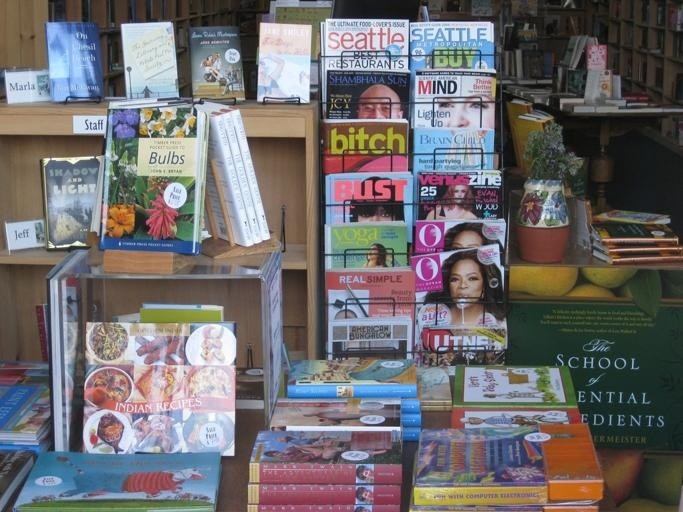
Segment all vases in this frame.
[515,179,571,263]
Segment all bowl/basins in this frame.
[83,367,134,410]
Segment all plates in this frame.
[183,411,236,453]
[133,413,183,454]
[185,323,237,367]
[83,408,133,454]
[246,368,264,376]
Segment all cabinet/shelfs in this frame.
[537,0,683,159]
[0,99,320,359]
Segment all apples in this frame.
[509,265,638,298]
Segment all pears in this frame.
[596,448,683,512]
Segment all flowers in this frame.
[523,123,585,180]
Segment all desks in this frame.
[214,410,616,512]
[504,94,683,214]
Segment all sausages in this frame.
[135,336,182,364]
